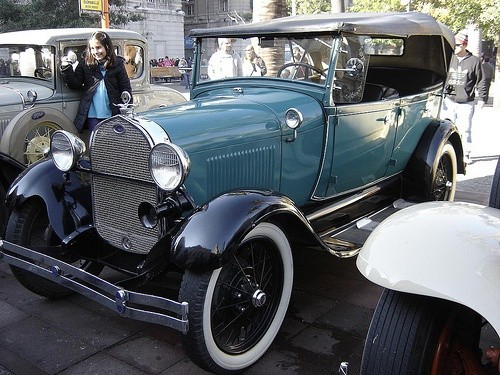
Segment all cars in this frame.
[0,27,192,170]
[1,10,500,374]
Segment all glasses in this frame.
[456,44,465,46]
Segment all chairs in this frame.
[332,80,390,107]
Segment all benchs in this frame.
[344,64,438,97]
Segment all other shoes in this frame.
[463,154,471,164]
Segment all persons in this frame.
[481,55,495,104]
[434,33,488,167]
[242,45,267,76]
[477,55,484,84]
[59,30,134,137]
[488,57,495,66]
[281,68,290,79]
[291,47,310,81]
[207,37,243,80]
[126,56,193,89]
[0,51,22,75]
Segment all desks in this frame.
[150,65,183,83]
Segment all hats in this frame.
[454,33,468,46]
[244,45,253,51]
